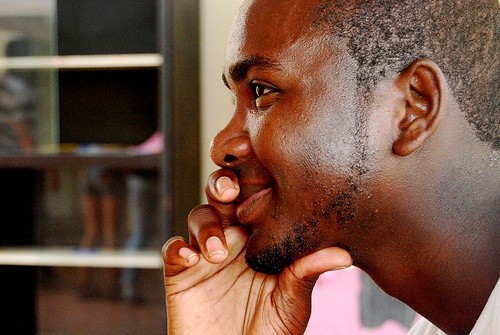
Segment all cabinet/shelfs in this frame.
[0,0,201,334]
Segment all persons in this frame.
[0,39,161,304]
[161,0,500,335]
